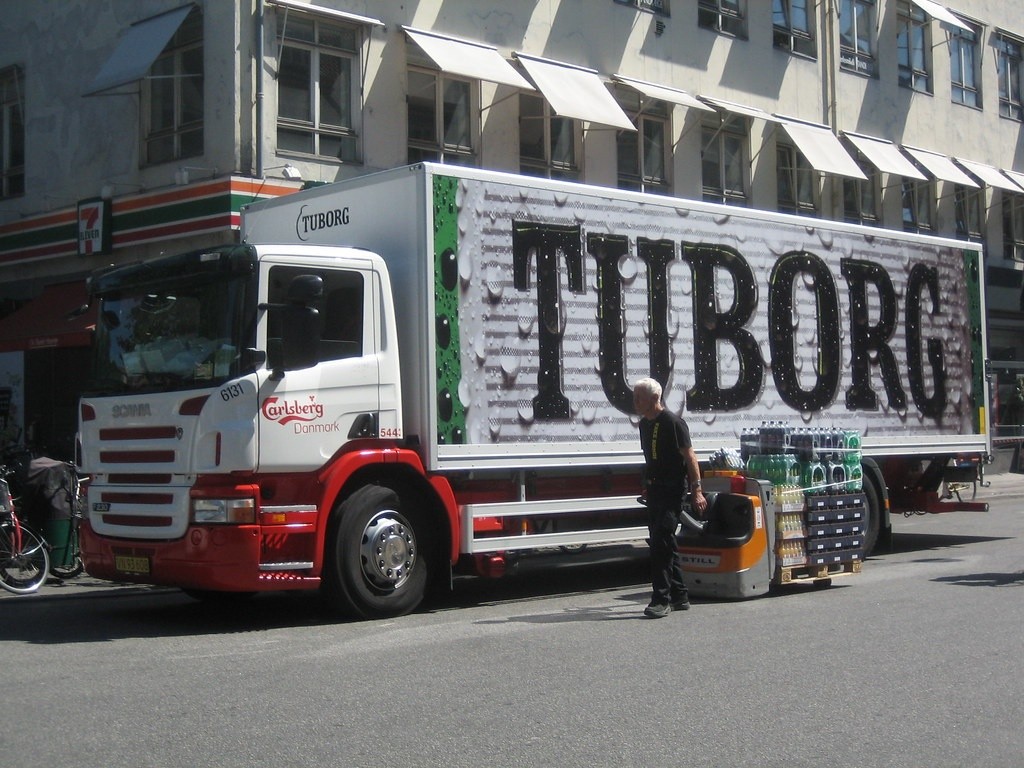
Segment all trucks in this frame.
[66,161,1000,620]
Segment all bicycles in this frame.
[938,478,980,502]
[0,461,51,594]
[48,463,94,580]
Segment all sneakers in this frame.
[671,601,690,611]
[644,603,672,617]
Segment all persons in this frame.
[633,378,708,618]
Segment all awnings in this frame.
[265,0,385,93]
[899,0,975,49]
[615,77,717,148]
[955,159,1024,211]
[901,147,981,201]
[776,116,869,181]
[515,56,638,131]
[844,134,930,189]
[81,7,203,96]
[1002,171,1024,214]
[404,28,536,112]
[703,98,788,163]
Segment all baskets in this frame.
[0,479,12,515]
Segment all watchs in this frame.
[692,480,702,487]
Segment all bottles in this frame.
[710,420,862,559]
